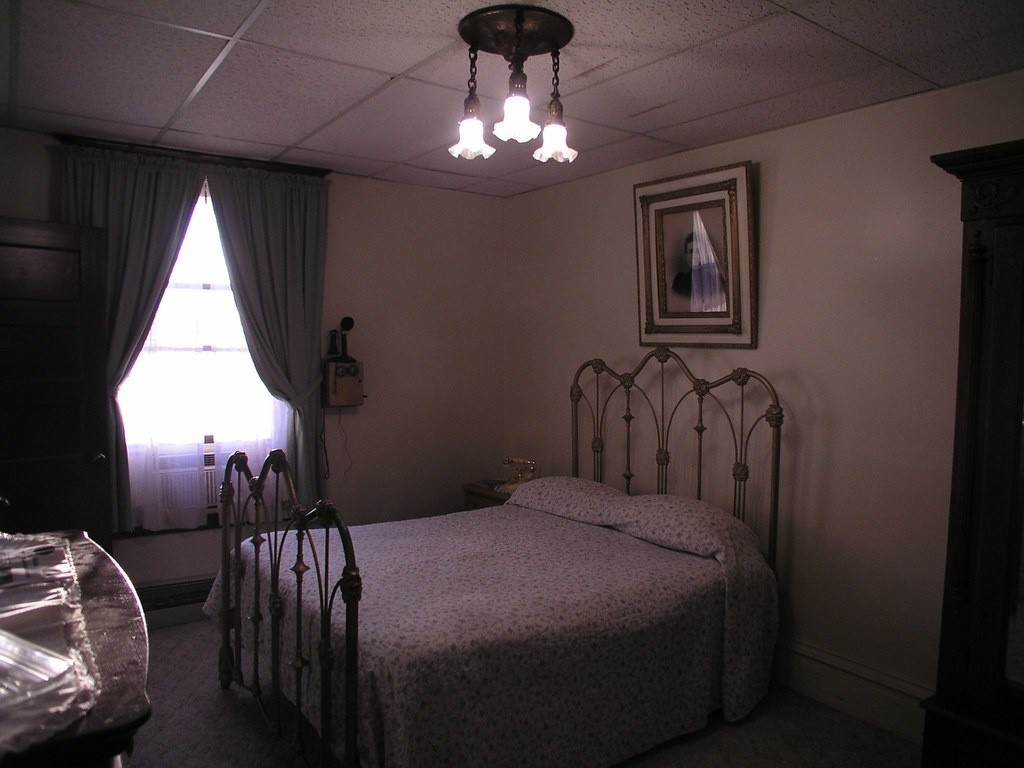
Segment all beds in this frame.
[201,344,786,768]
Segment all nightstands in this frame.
[459,480,510,510]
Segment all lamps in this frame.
[448,3,579,164]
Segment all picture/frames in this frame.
[632,160,758,352]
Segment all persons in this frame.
[671,232,725,298]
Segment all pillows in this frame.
[510,477,785,725]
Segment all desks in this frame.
[7,527,153,768]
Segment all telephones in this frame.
[503,457,536,492]
